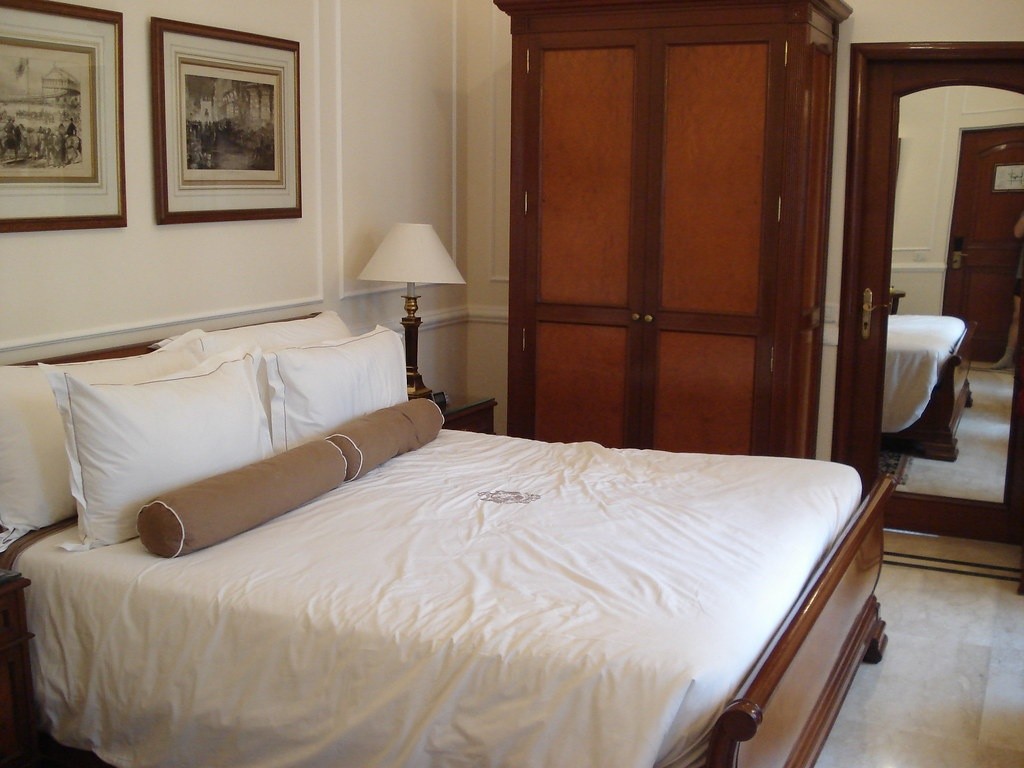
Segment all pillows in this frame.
[0,311,445,558]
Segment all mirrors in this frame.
[872,79,1024,510]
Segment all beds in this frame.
[881,315,978,463]
[0,312,899,768]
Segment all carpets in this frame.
[872,450,913,484]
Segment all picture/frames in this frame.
[0,0,127,233]
[151,17,302,225]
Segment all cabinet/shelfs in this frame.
[437,393,498,435]
[493,0,853,459]
[0,569,52,768]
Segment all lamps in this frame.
[357,222,466,401]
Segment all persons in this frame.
[991,211,1024,368]
